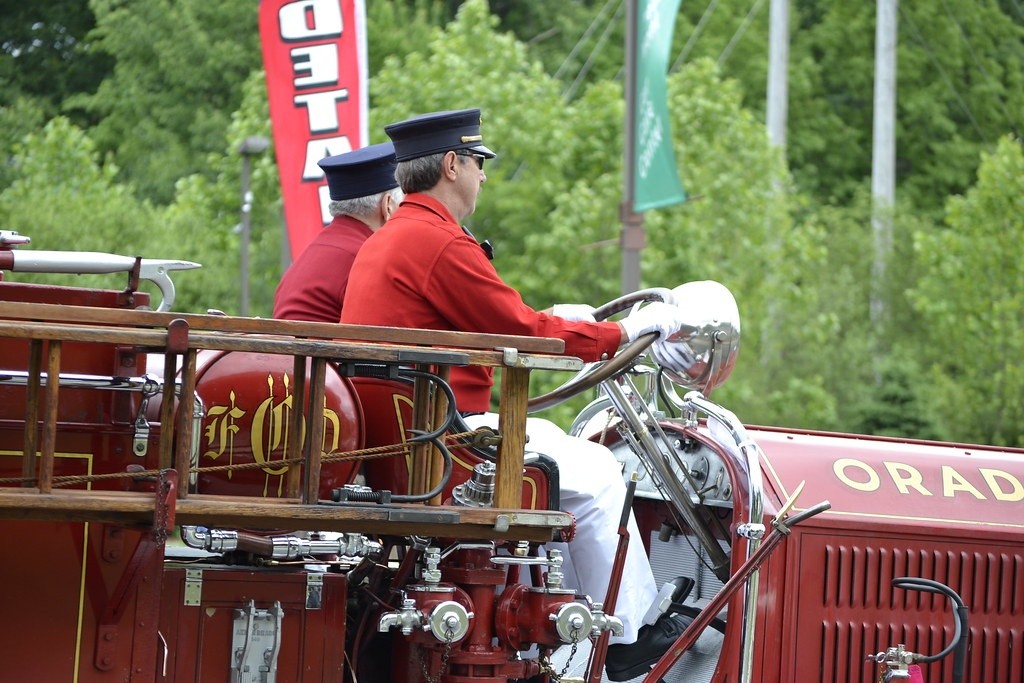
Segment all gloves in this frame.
[553,304,607,323]
[619,301,682,344]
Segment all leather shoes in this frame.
[605,607,702,681]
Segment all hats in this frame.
[317,142,400,201]
[383,107,496,162]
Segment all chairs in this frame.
[333,363,558,509]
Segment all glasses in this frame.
[442,153,485,169]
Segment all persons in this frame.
[338,106,698,682]
[272,145,406,322]
